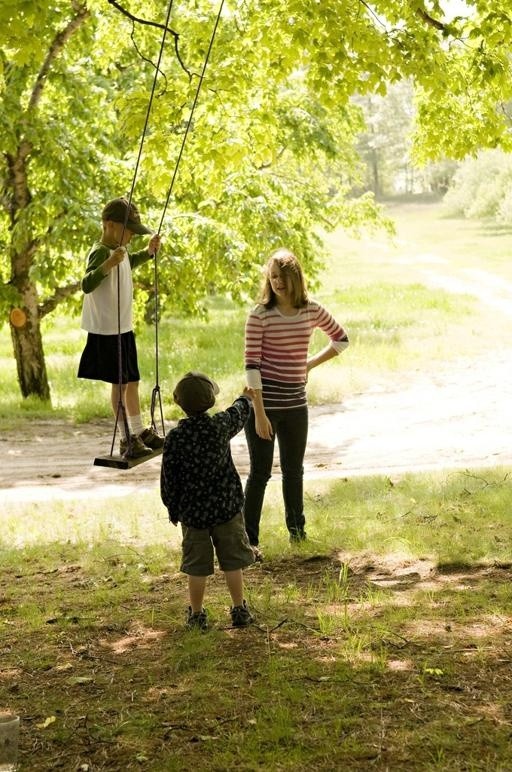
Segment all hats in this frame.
[101,196,153,235]
[172,370,220,413]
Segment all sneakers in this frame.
[229,598,254,626]
[119,428,166,460]
[187,605,208,630]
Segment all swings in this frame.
[94,0,226,468]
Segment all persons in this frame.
[242,250,349,559]
[161,372,257,630]
[78,197,165,459]
